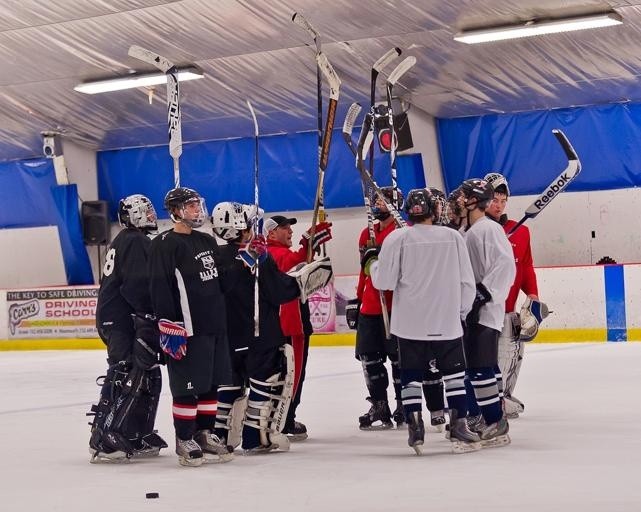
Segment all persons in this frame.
[86,193,168,462]
[146,187,235,467]
[211,202,334,456]
[360,189,482,455]
[260,215,333,443]
[346,186,408,429]
[430,174,549,447]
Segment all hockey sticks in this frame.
[243,98,259,335]
[291,13,326,225]
[342,48,422,340]
[306,53,342,264]
[126,46,182,189]
[507,128,579,240]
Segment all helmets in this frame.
[118,187,264,241]
[370,172,510,220]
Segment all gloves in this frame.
[360,246,381,276]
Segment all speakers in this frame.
[373,112,414,153]
[42,134,62,159]
[81,201,112,246]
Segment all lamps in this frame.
[74,65,205,95]
[453,10,624,45]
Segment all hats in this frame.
[262,216,297,238]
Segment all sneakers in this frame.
[176,430,234,458]
[359,396,509,446]
[283,422,306,434]
